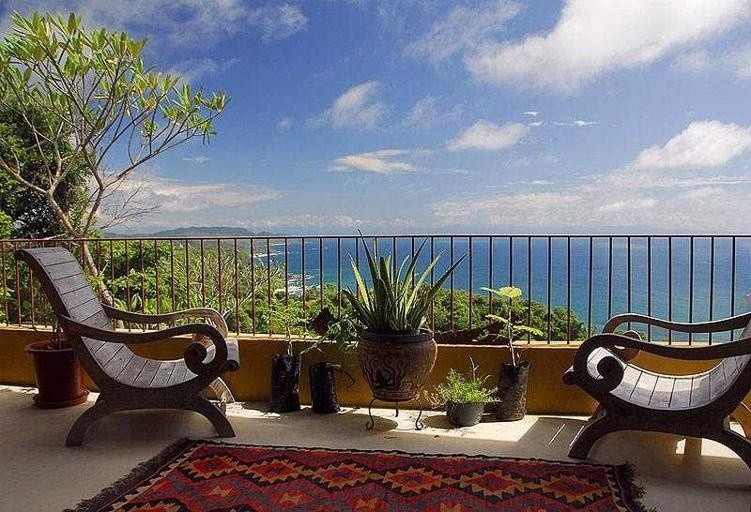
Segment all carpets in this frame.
[66,437,649,512]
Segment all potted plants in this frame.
[268,307,358,414]
[424,286,545,428]
[340,228,468,400]
[23,272,112,409]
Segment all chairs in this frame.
[562,311,751,472]
[13,245,240,447]
